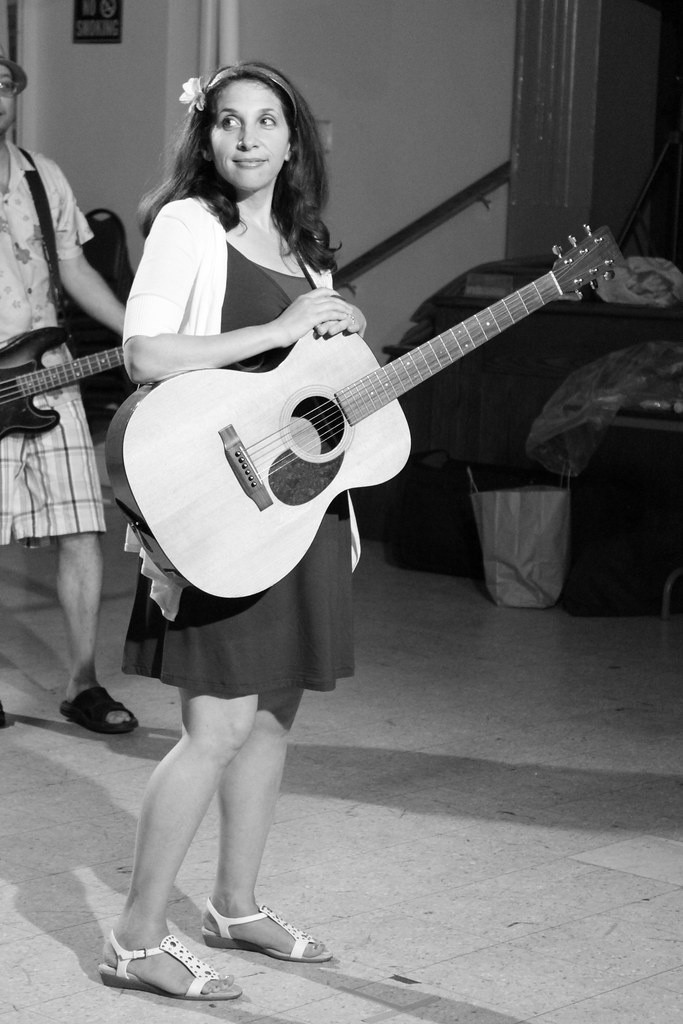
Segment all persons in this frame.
[0,51,142,736]
[99,61,367,1001]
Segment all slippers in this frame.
[60,685,138,734]
[0,700,6,728]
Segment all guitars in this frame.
[103,218,628,611]
[0,322,124,442]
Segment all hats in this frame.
[0,44,27,95]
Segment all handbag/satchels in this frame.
[467,453,573,608]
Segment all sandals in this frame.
[97,926,243,1001]
[201,894,333,962]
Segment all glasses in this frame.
[0,81,21,98]
